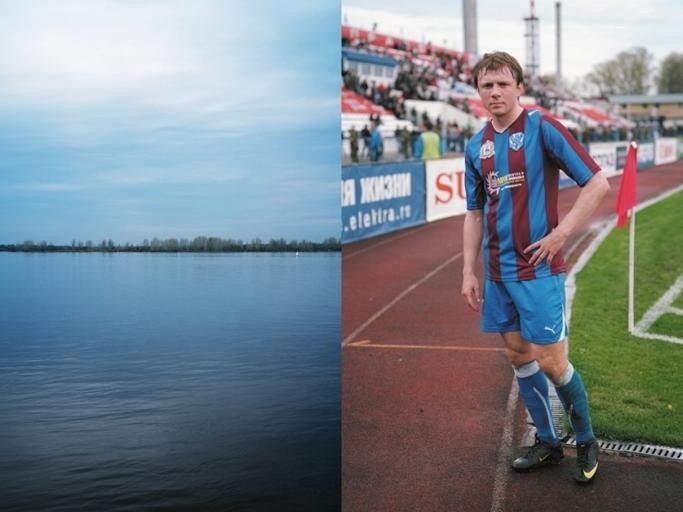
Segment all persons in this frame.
[341,37,683,162]
[461,53,610,485]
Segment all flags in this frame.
[614,146,637,228]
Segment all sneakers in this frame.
[573,438,599,485]
[512,434,564,472]
